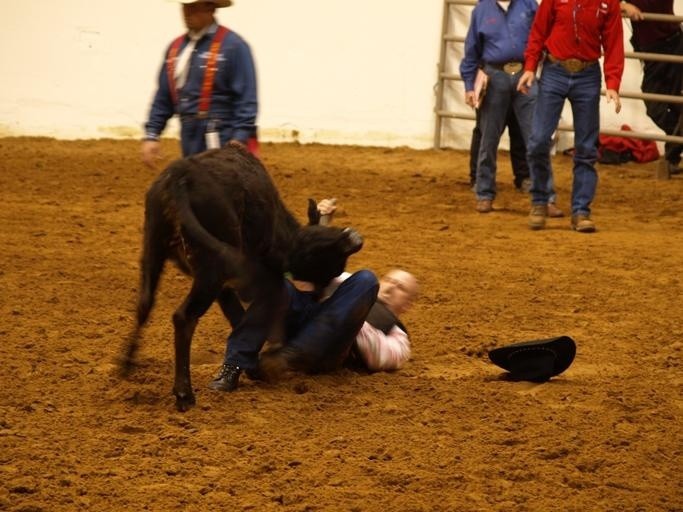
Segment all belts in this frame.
[487,60,525,75]
[547,53,598,74]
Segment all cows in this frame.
[115,148,358,413]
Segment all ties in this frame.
[169,29,205,91]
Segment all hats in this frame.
[170,1,234,9]
[485,335,578,383]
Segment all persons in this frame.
[203,196,420,393]
[468,81,530,193]
[139,0,261,178]
[618,1,682,175]
[513,1,625,233]
[457,0,563,219]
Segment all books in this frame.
[471,68,491,111]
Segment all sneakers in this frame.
[545,200,565,218]
[526,201,549,228]
[212,360,241,393]
[474,194,493,212]
[570,207,597,233]
